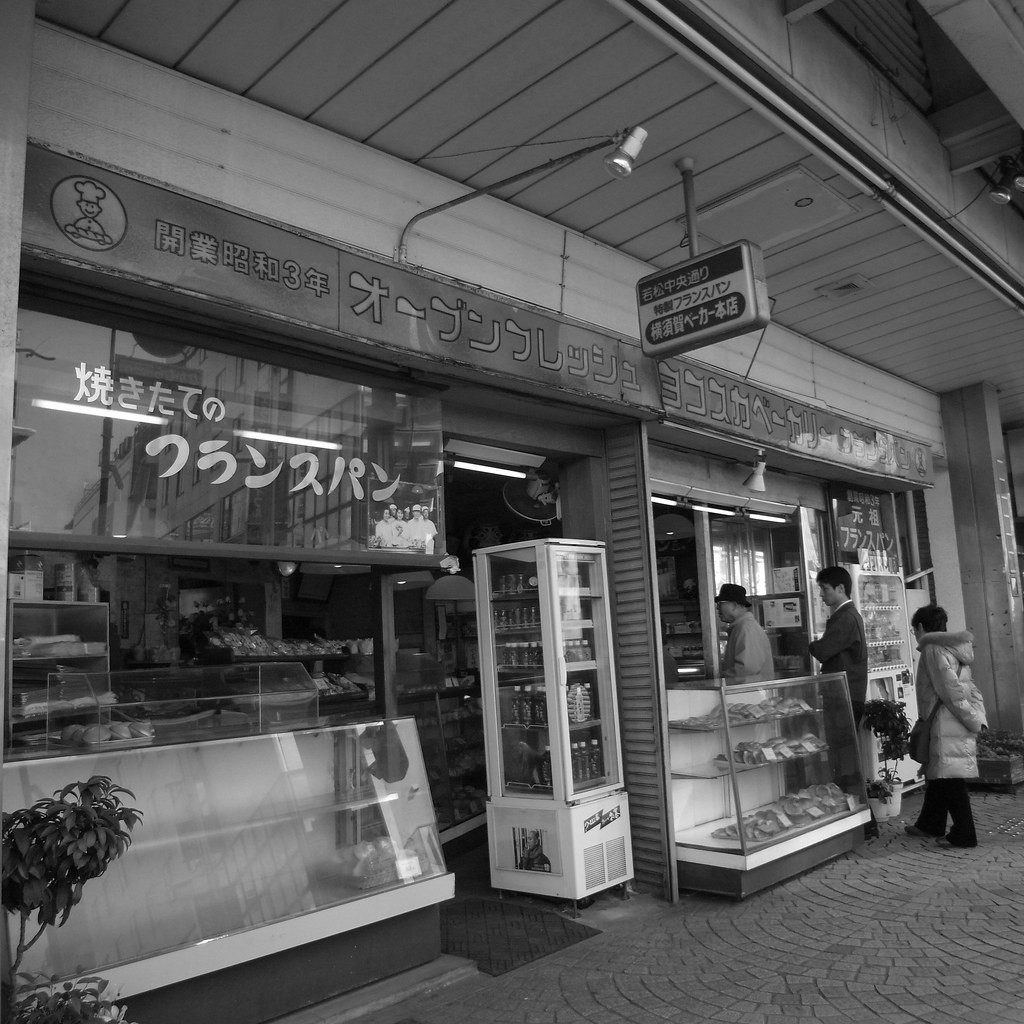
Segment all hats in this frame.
[714,584,752,607]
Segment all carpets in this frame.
[439,898,603,978]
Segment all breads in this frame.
[682,696,851,840]
[63,724,152,743]
[393,681,487,831]
[337,836,393,878]
[209,632,342,656]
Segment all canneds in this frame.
[496,573,537,627]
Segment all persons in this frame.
[519,830,552,872]
[374,504,439,554]
[904,605,987,847]
[808,565,880,840]
[714,583,775,677]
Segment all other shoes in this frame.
[936,838,959,847]
[904,825,945,836]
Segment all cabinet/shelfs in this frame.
[6,598,113,721]
[665,618,728,662]
[207,630,483,804]
[669,708,832,783]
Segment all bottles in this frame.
[511,682,595,726]
[542,739,600,786]
[859,547,888,572]
[503,640,592,669]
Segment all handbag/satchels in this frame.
[909,719,931,765]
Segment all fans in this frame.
[501,472,561,527]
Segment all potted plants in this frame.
[866,776,893,823]
[861,699,912,817]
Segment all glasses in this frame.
[716,600,730,606]
[910,628,917,635]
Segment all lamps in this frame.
[987,157,1024,205]
[742,449,768,494]
[396,125,649,265]
[278,539,475,601]
